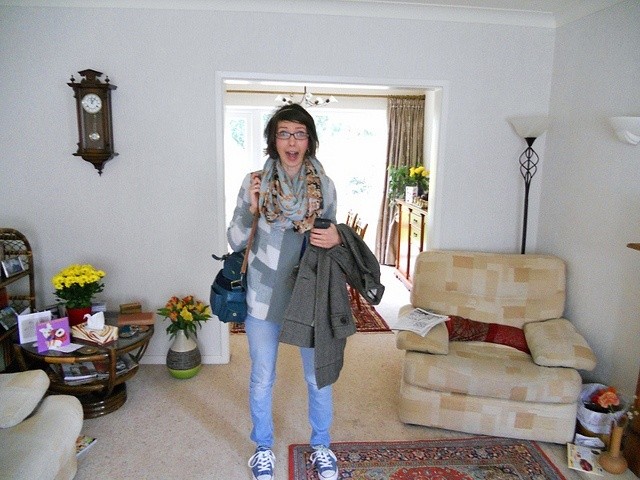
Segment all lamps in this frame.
[273,86,337,109]
[510,116,547,255]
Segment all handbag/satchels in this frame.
[210,250,261,324]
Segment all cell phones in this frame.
[309,214,332,250]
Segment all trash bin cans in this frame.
[575,383,624,450]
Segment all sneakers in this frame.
[247,449,276,480]
[309,446,338,479]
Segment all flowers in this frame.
[387,165,429,198]
[156,295,211,340]
[52,263,105,309]
[598,390,640,425]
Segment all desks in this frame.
[14,301,155,420]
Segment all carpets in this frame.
[288,436,569,479]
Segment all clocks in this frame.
[67,68,120,177]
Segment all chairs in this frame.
[349,218,368,311]
[344,210,358,228]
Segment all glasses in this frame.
[274,131,310,141]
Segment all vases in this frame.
[166,330,201,379]
[599,425,628,474]
[66,309,92,326]
[405,186,418,204]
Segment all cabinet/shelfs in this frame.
[0,227,36,374]
[396,204,427,288]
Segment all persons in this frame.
[225,103,343,480]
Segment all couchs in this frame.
[396,251,597,445]
[0,370,85,480]
